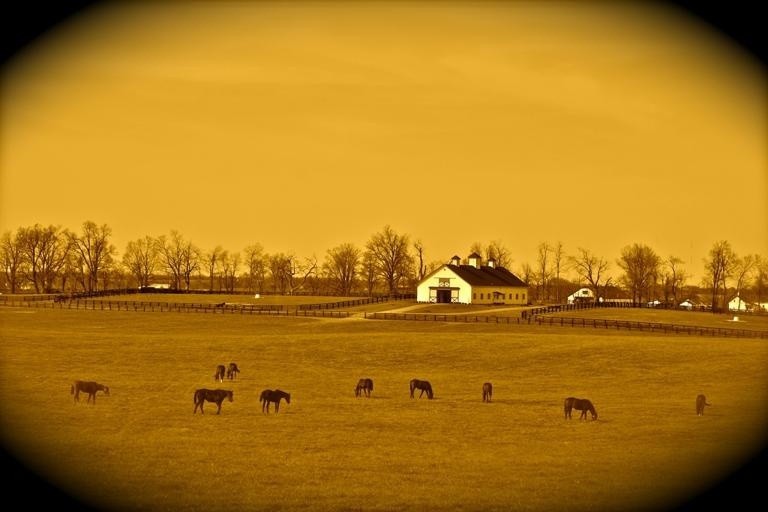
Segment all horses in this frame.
[694,394,713,415]
[259,389,292,414]
[69,379,109,405]
[212,364,225,383]
[480,382,494,402]
[191,388,234,414]
[562,396,599,420]
[226,362,241,379]
[353,378,374,397]
[408,378,434,400]
